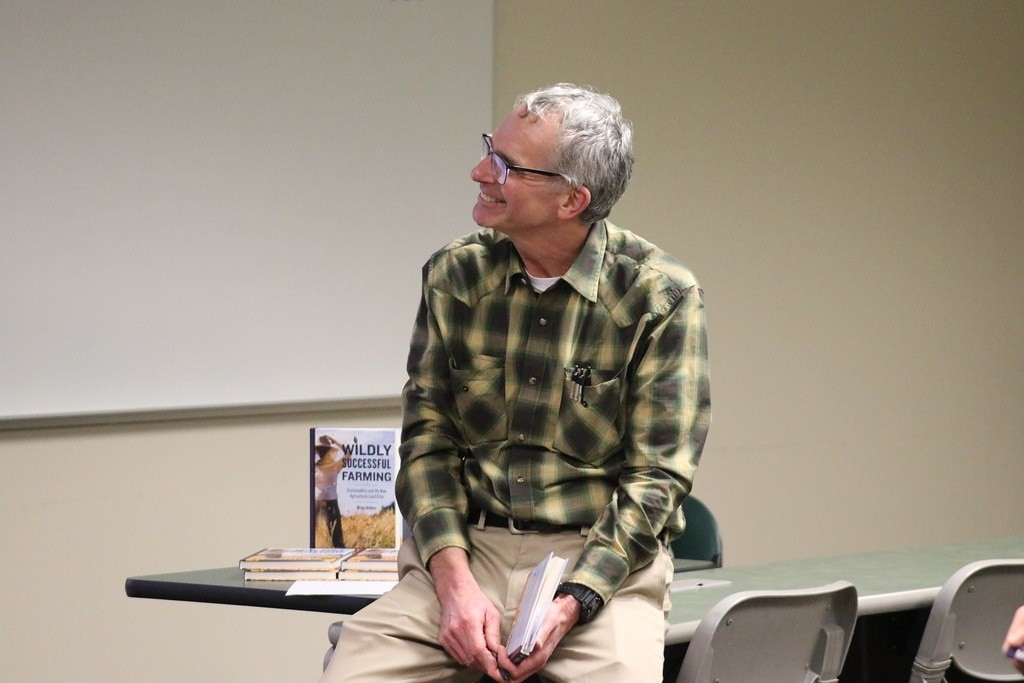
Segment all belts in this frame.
[467,508,591,534]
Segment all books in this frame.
[239,547,400,582]
[505,552,569,665]
[310,426,400,550]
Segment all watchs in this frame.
[556,583,602,625]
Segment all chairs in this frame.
[680,580,860,683]
[670,495,723,567]
[909,558,1024,683]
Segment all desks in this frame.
[125,535,1024,675]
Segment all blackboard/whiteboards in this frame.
[1,0,497,425]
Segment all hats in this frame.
[315,435,341,450]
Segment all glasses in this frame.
[481,133,561,185]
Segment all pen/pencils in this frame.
[569,364,591,408]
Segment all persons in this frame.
[325,82,710,683]
[315,435,350,548]
